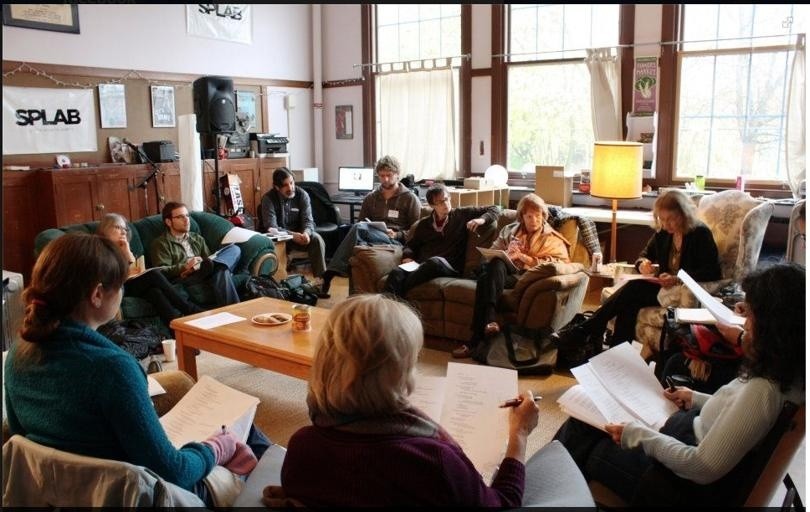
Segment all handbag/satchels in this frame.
[559,314,603,369]
[96,319,166,359]
[246,274,318,306]
[663,305,745,361]
[487,332,558,374]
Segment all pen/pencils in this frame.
[365,218,373,222]
[499,395,544,408]
[665,376,676,395]
[221,425,227,433]
[474,232,481,238]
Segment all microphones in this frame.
[123,137,138,151]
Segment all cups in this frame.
[161,339,177,361]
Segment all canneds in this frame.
[291,304,312,332]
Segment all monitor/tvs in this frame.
[338,166,375,197]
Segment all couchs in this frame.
[33,211,278,321]
[348,204,590,353]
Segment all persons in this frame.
[300,156,422,298]
[261,166,328,279]
[4,232,273,507]
[149,201,242,306]
[96,213,207,355]
[551,260,805,509]
[452,192,572,359]
[383,182,501,297]
[261,294,539,511]
[716,199,806,348]
[551,189,723,351]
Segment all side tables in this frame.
[583,269,614,346]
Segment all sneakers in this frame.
[300,280,329,294]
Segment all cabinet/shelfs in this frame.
[133,167,180,219]
[224,162,275,217]
[58,173,131,227]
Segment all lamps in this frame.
[589,141,642,264]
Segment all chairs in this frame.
[596,189,774,350]
[0,372,206,508]
[521,402,805,511]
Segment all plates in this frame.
[251,312,291,327]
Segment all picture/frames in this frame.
[3,4,80,35]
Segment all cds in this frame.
[56,155,71,167]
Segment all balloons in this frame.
[483,164,510,189]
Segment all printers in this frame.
[249,132,288,153]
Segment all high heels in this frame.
[552,326,592,345]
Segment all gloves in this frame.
[203,431,258,475]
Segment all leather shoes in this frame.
[453,344,474,357]
[486,322,500,335]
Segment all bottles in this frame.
[695,175,705,192]
[218,148,225,160]
[579,170,591,193]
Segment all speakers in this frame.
[192,75,236,137]
